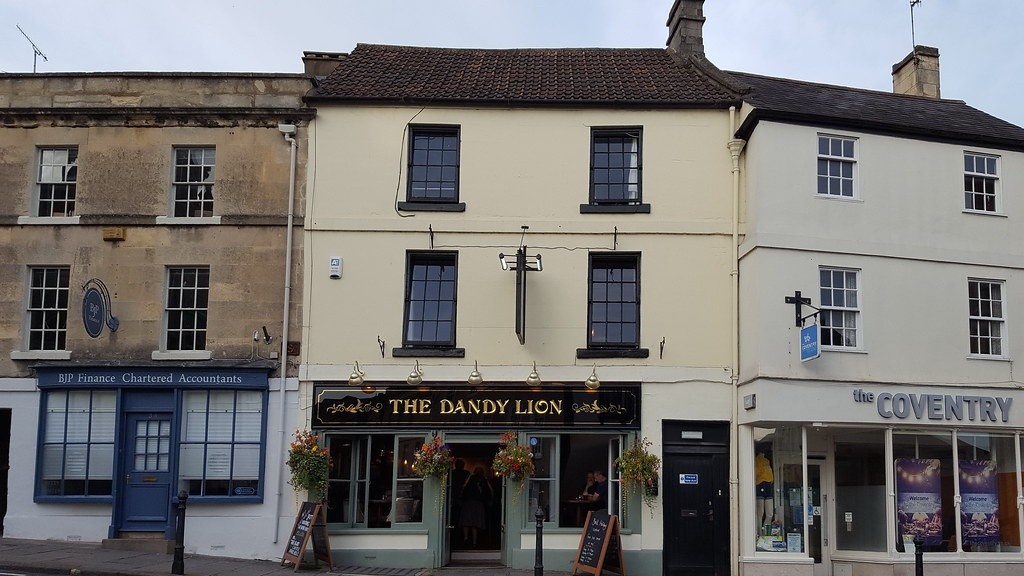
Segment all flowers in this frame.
[491,430,535,506]
[613,437,661,519]
[286,428,333,512]
[413,434,456,510]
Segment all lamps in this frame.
[348,360,365,386]
[526,361,541,386]
[407,360,423,385]
[585,363,600,389]
[468,360,483,384]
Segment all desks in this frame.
[568,500,590,526]
[369,500,389,521]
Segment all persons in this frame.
[448,458,502,548]
[578,471,608,516]
[754,453,773,536]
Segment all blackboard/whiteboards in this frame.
[573,511,625,573]
[282,501,334,563]
